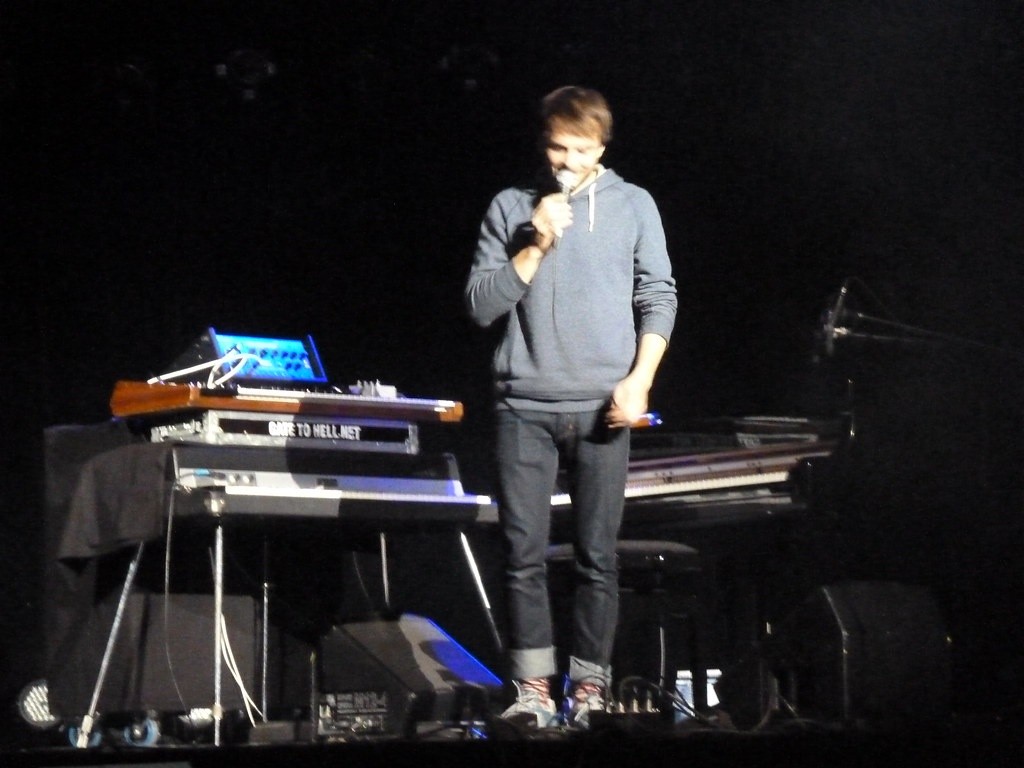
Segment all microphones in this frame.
[551,170,574,250]
[823,285,850,350]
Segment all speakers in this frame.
[715,579,951,727]
[47,578,265,724]
[311,614,505,743]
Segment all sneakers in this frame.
[501,678,558,729]
[561,695,613,731]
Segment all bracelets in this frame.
[532,241,546,254]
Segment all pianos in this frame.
[108,377,465,426]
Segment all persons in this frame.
[462,86,678,729]
